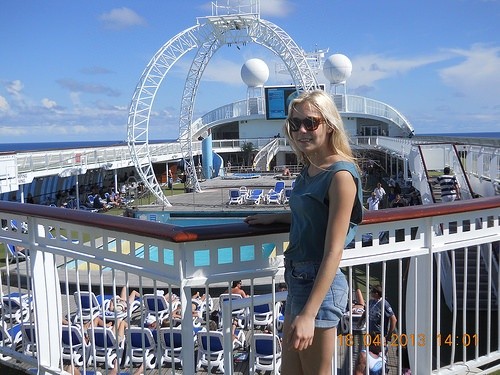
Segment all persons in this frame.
[11,160,232,256]
[279,90,363,375]
[366,161,461,224]
[282,166,291,177]
[60,279,397,375]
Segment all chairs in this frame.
[0,288,285,375]
[6,191,134,261]
[228,180,295,205]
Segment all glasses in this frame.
[288,116,327,132]
[238,282,241,285]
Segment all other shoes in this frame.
[233,317,239,326]
